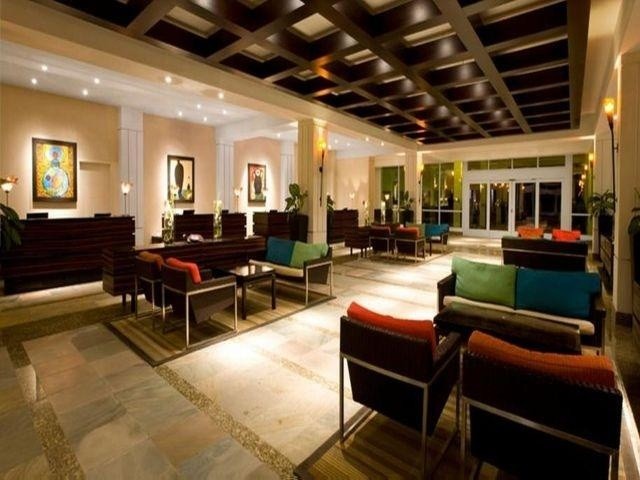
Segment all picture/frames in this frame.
[248,163,267,202]
[32,137,77,203]
[167,155,195,203]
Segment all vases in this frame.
[212,199,224,238]
[163,200,175,245]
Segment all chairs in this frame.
[132,250,238,350]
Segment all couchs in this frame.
[248,235,333,309]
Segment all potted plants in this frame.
[627,186,640,287]
[586,189,617,238]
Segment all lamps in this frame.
[589,152,596,180]
[600,97,619,153]
[233,182,243,213]
[119,180,134,215]
[0,176,18,207]
[348,191,355,207]
